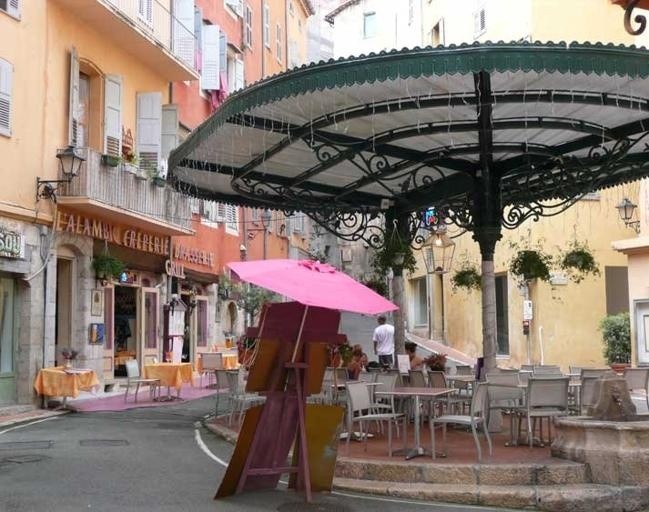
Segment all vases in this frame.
[64,358,72,369]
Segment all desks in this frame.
[114,351,136,376]
[39,366,95,412]
[373,386,457,460]
[145,362,192,402]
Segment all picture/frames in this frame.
[89,323,105,345]
[90,288,103,317]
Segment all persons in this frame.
[405,341,424,369]
[344,344,377,379]
[373,314,396,364]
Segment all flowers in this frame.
[59,347,80,359]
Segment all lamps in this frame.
[35,138,86,203]
[246,208,273,231]
[614,197,640,233]
[421,222,455,275]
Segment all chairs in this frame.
[430,382,491,462]
[307,364,641,449]
[198,352,268,430]
[123,359,161,404]
[345,380,408,461]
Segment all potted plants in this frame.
[102,148,166,189]
[449,255,481,298]
[502,235,602,302]
[89,253,126,281]
[368,228,419,281]
[596,311,631,372]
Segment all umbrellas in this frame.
[225,259,399,392]
